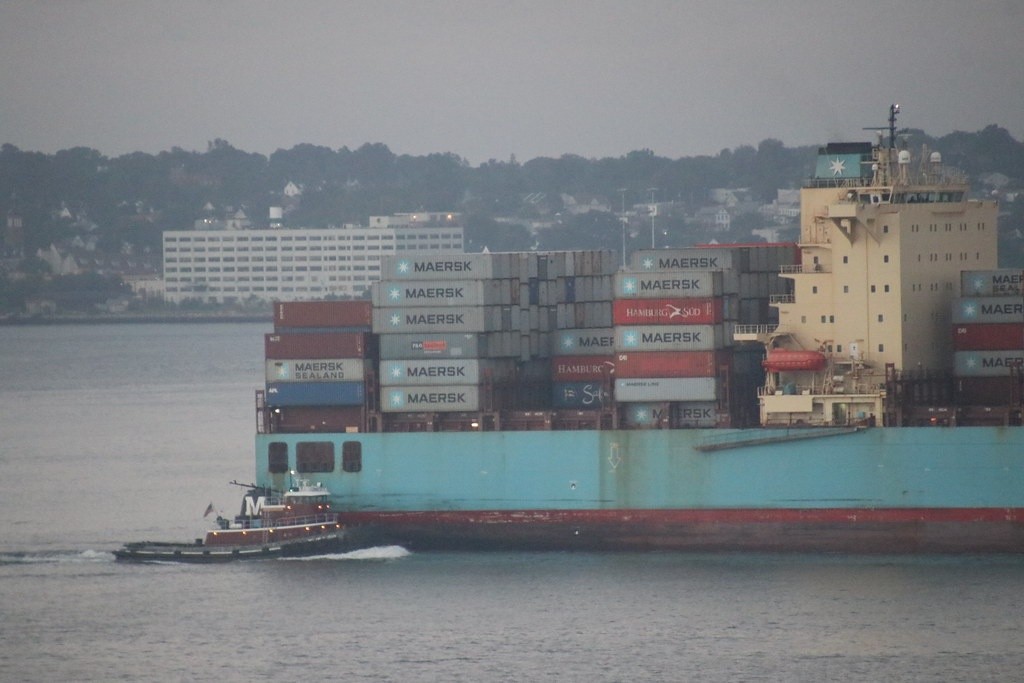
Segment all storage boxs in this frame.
[261,241,1024,433]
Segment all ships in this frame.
[255,104,1023,558]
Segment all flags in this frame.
[204,504,214,518]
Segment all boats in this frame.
[112,469,350,564]
[760,348,828,371]
